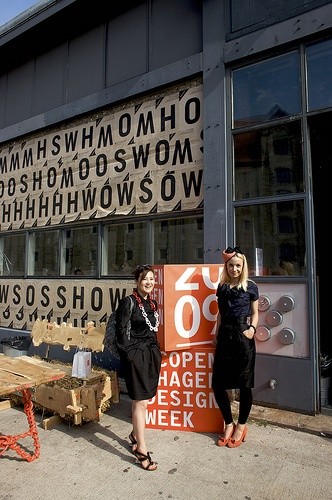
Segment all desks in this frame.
[0,355,65,463]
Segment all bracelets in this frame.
[249,325,256,334]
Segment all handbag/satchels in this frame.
[102,295,134,361]
[71,351,91,379]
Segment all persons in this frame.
[272,256,297,275]
[211,244,259,448]
[121,260,135,273]
[73,268,84,275]
[114,264,162,471]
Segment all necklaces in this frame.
[132,291,159,332]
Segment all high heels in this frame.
[227,423,248,448]
[128,431,138,453]
[133,449,158,471]
[217,423,236,447]
